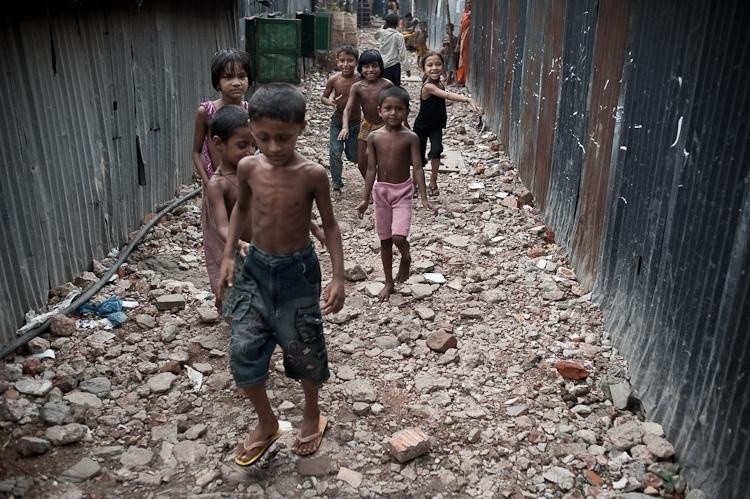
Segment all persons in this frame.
[373,0,473,87]
[412,52,482,197]
[320,47,440,304]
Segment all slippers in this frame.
[291,415,328,456]
[428,184,440,196]
[233,431,283,466]
